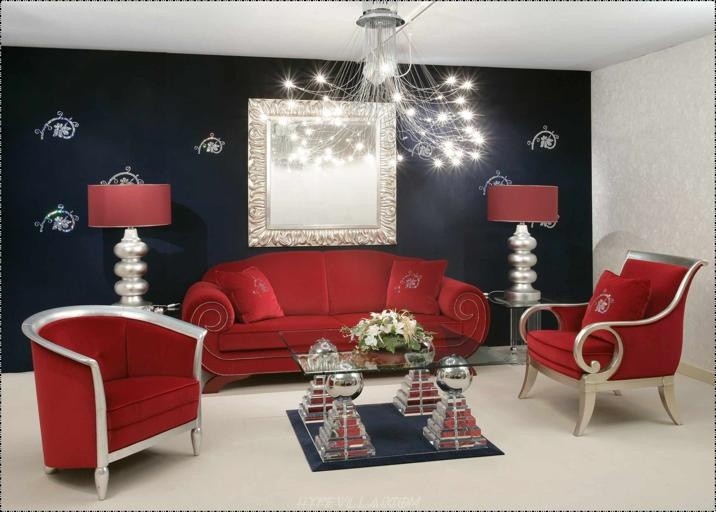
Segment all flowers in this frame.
[337,304,438,356]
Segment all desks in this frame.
[484,291,561,355]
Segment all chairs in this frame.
[24,301,210,503]
[517,248,712,438]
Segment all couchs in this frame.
[180,244,498,397]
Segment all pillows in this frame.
[582,266,653,345]
[214,261,288,324]
[385,255,447,318]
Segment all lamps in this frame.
[484,181,565,303]
[273,2,488,177]
[86,182,176,313]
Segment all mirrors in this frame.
[243,96,400,249]
